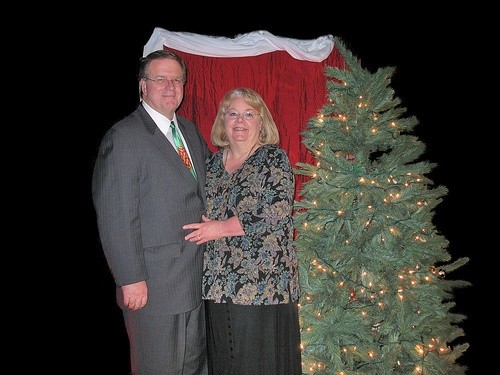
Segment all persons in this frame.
[91,50,213,375]
[183,86,302,375]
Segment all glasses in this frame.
[144,77,185,86]
[223,110,260,120]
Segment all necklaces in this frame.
[224,143,257,168]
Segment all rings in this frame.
[198,236,201,241]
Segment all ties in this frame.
[169,121,197,180]
